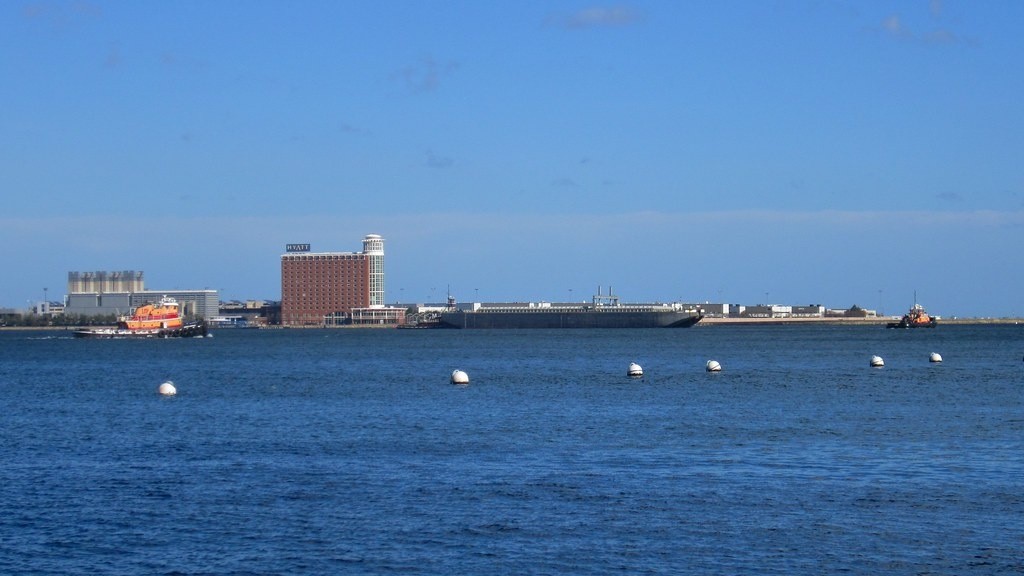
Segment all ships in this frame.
[439,308,705,330]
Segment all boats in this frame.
[886,290,937,329]
[72,294,209,338]
